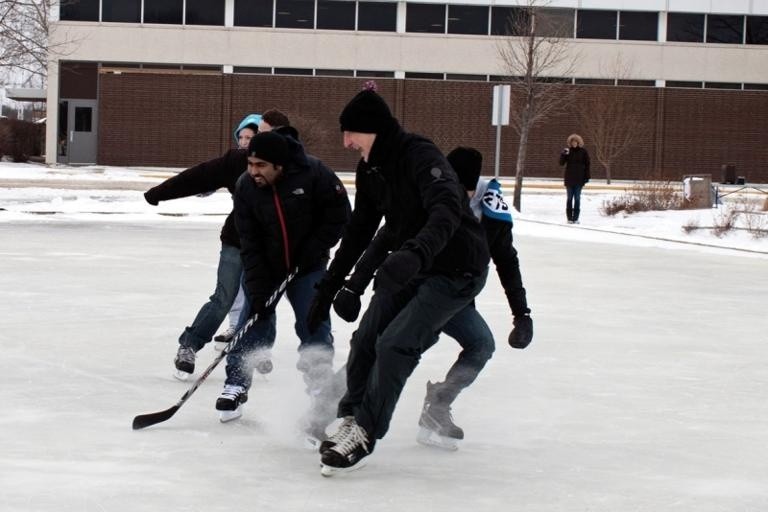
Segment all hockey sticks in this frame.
[133,268,300,428]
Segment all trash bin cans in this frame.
[682,174,713,209]
[720,162,736,184]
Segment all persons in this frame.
[419,144,533,435]
[216,123,260,341]
[559,132,592,224]
[311,86,489,468]
[145,106,298,374]
[214,133,353,410]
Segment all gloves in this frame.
[508,316,533,349]
[371,247,422,296]
[246,278,277,315]
[143,187,160,207]
[306,279,339,330]
[333,283,365,323]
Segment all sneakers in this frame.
[213,327,238,342]
[255,359,273,375]
[318,415,353,453]
[320,425,376,469]
[174,345,196,373]
[293,390,347,443]
[216,383,249,410]
[418,382,464,439]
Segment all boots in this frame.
[566,208,573,224]
[573,208,580,224]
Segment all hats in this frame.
[269,126,304,149]
[443,147,482,191]
[247,131,290,167]
[338,89,400,135]
